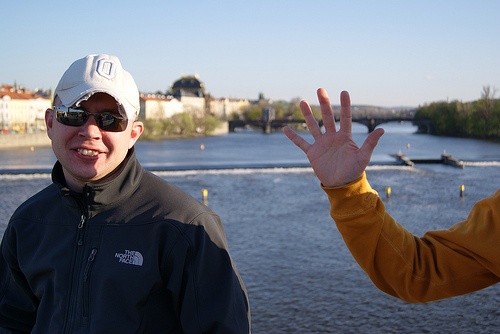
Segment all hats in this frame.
[52,54,141,120]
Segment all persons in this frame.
[282,87,500,303]
[0,55,251,334]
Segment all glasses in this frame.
[53,103,128,132]
